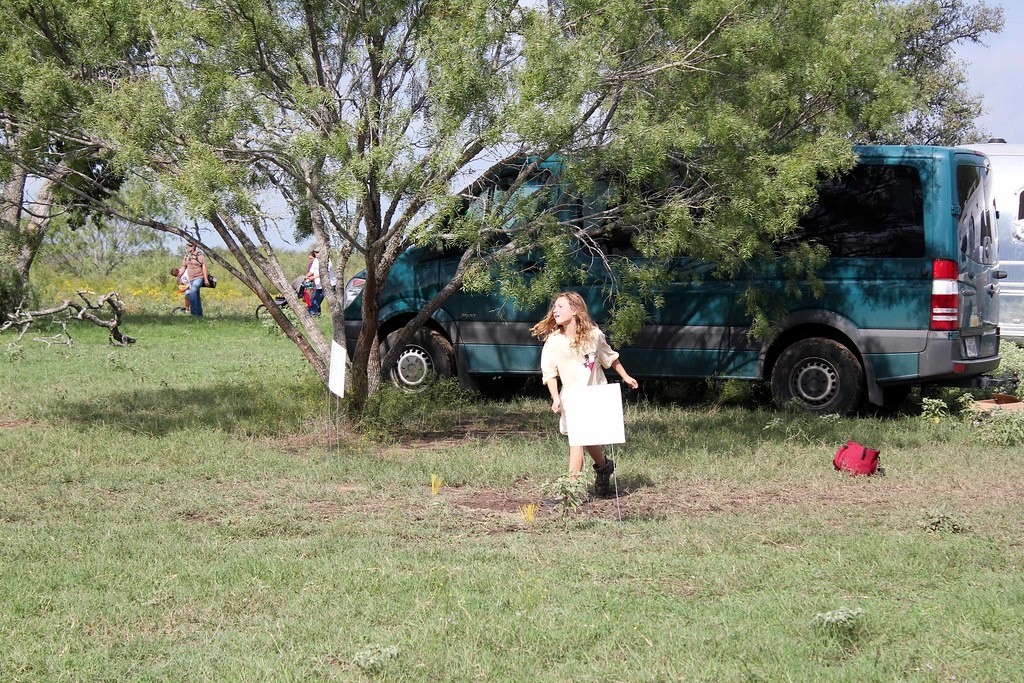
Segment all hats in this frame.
[314,246,322,252]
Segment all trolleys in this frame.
[256,277,313,320]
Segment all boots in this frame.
[591,455,616,496]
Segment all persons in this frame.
[527,291,640,497]
[304,251,317,291]
[307,247,339,317]
[171,268,193,311]
[174,241,210,315]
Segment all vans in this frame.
[345,144,1006,413]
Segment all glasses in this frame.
[186,243,194,247]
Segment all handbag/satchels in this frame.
[199,274,217,288]
[832,441,880,476]
